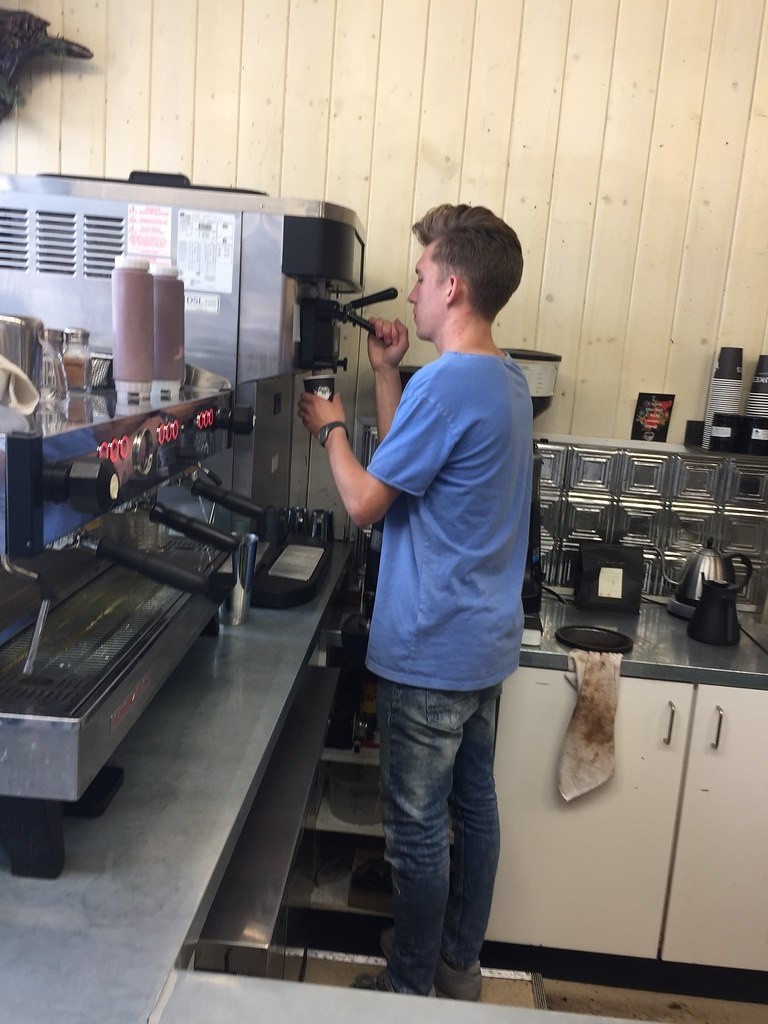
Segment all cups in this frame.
[702,347,743,450]
[0,314,41,398]
[289,507,308,535]
[745,355,768,417]
[311,510,333,541]
[219,531,258,626]
[303,375,335,401]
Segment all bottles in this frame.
[63,329,91,392]
[355,689,378,752]
[44,328,66,397]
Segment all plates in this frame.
[557,626,633,653]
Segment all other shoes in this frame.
[380,925,482,1002]
[348,972,399,993]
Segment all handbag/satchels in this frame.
[574,541,644,612]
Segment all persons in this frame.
[298,204,534,1001]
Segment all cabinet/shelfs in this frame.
[480,667,768,1003]
[280,745,455,928]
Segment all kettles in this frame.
[653,537,752,605]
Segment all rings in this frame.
[302,414,307,420]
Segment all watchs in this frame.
[317,421,349,447]
